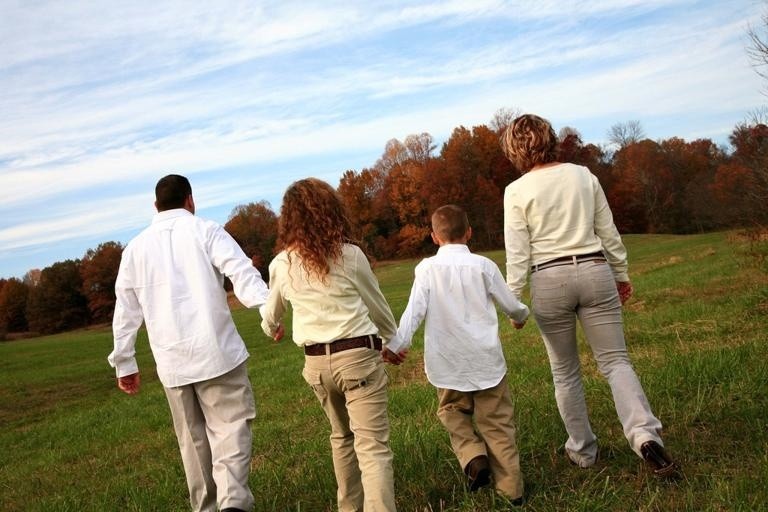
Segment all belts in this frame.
[528,250,608,273]
[303,333,384,357]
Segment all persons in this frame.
[261,179,406,512]
[504,115,671,473]
[108,175,284,510]
[382,204,530,504]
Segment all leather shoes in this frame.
[640,440,677,479]
[464,454,491,496]
